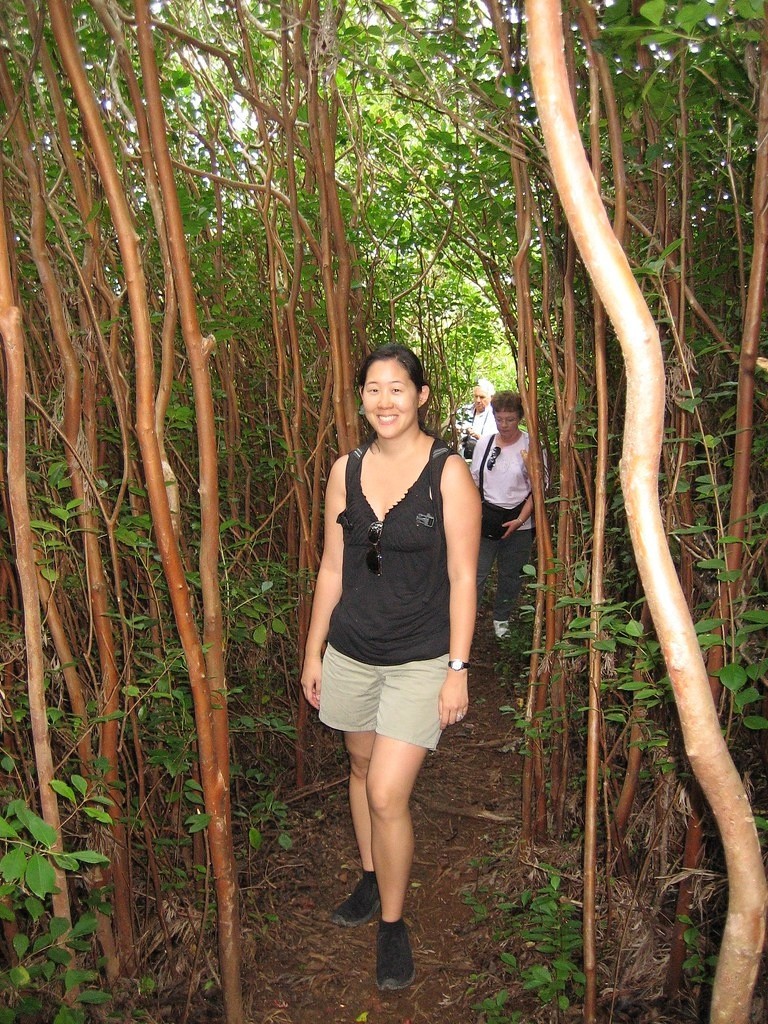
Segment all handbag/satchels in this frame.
[464,437,477,459]
[480,499,518,541]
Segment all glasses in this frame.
[365,521,384,576]
[487,447,501,471]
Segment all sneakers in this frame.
[376,926,416,991]
[329,877,380,929]
[493,619,511,638]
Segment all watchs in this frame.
[448,659,469,670]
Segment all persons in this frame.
[469,390,550,642]
[452,379,498,468]
[301,344,483,987]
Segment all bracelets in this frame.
[516,518,525,526]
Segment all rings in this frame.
[457,715,463,718]
